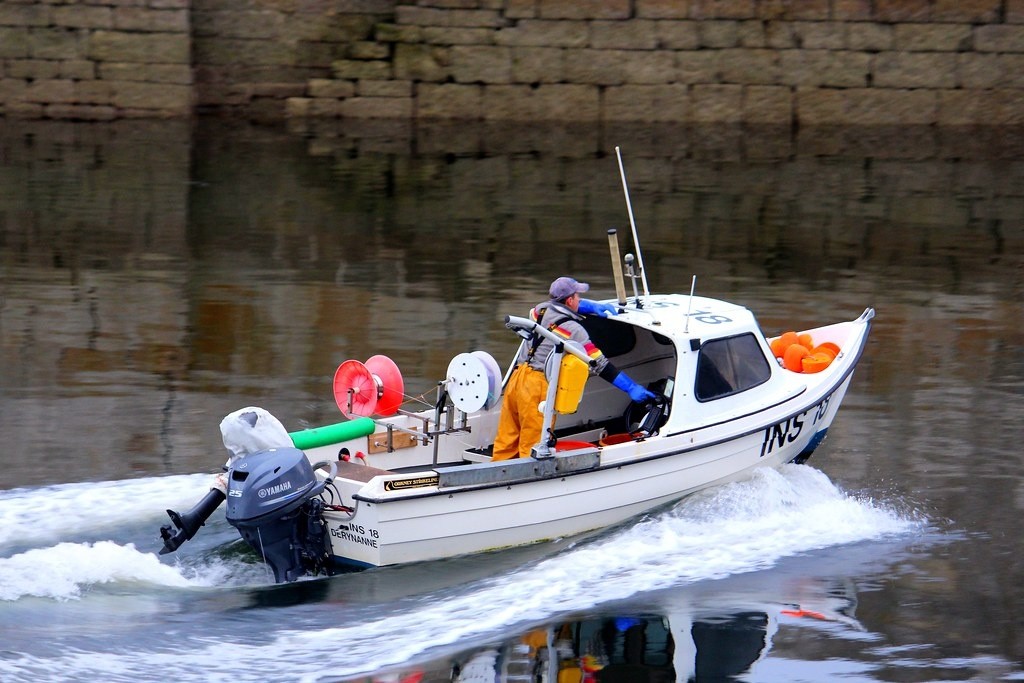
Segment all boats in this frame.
[160,146,876,584]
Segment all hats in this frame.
[548,276,590,302]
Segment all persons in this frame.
[489,276,656,462]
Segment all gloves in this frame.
[612,370,656,404]
[577,298,618,317]
